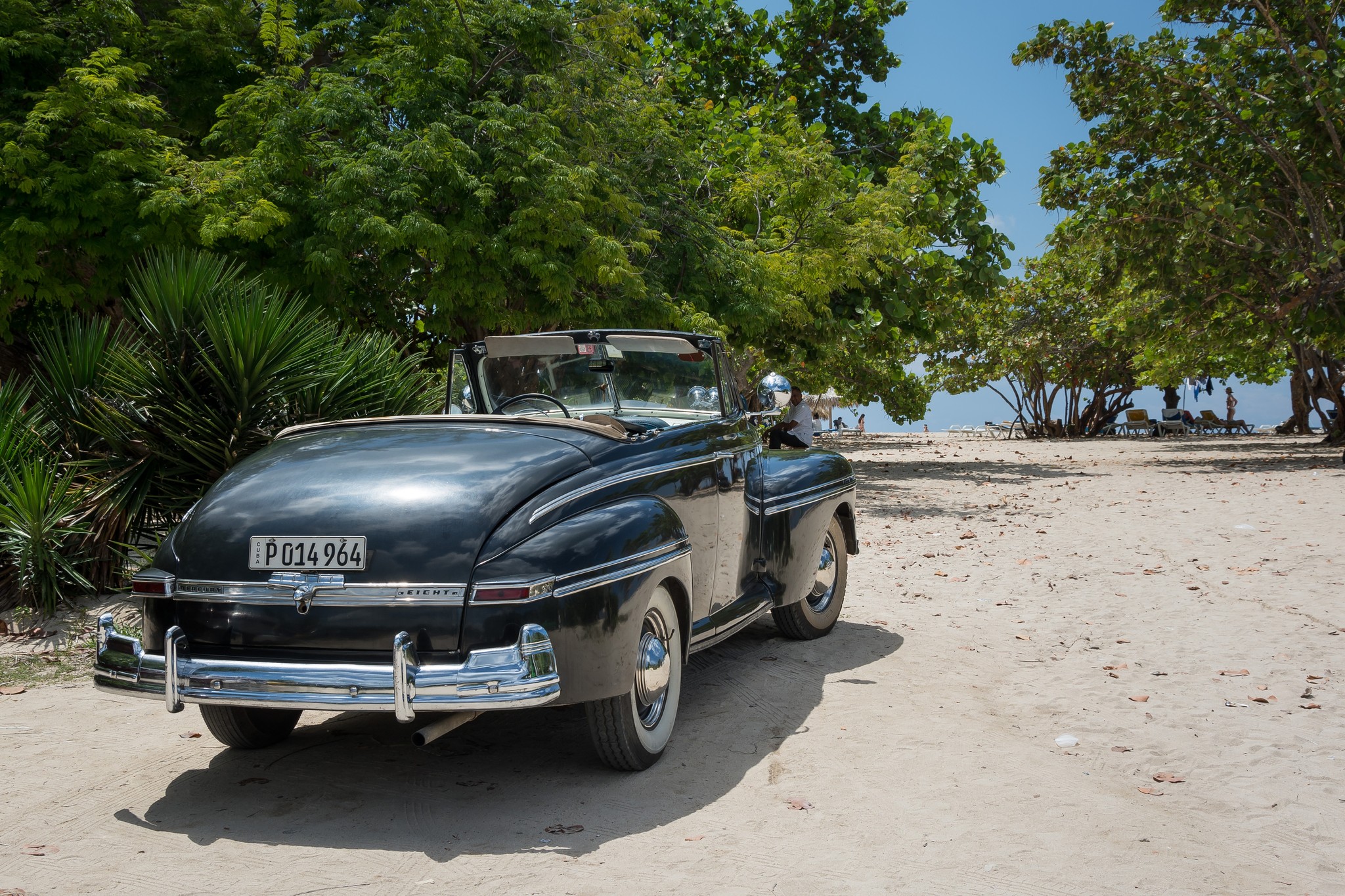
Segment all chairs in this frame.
[754,419,771,428]
[941,408,1300,440]
[1320,409,1338,434]
[810,420,863,439]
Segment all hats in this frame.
[838,417,843,420]
[1224,387,1234,393]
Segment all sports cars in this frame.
[92,327,858,775]
